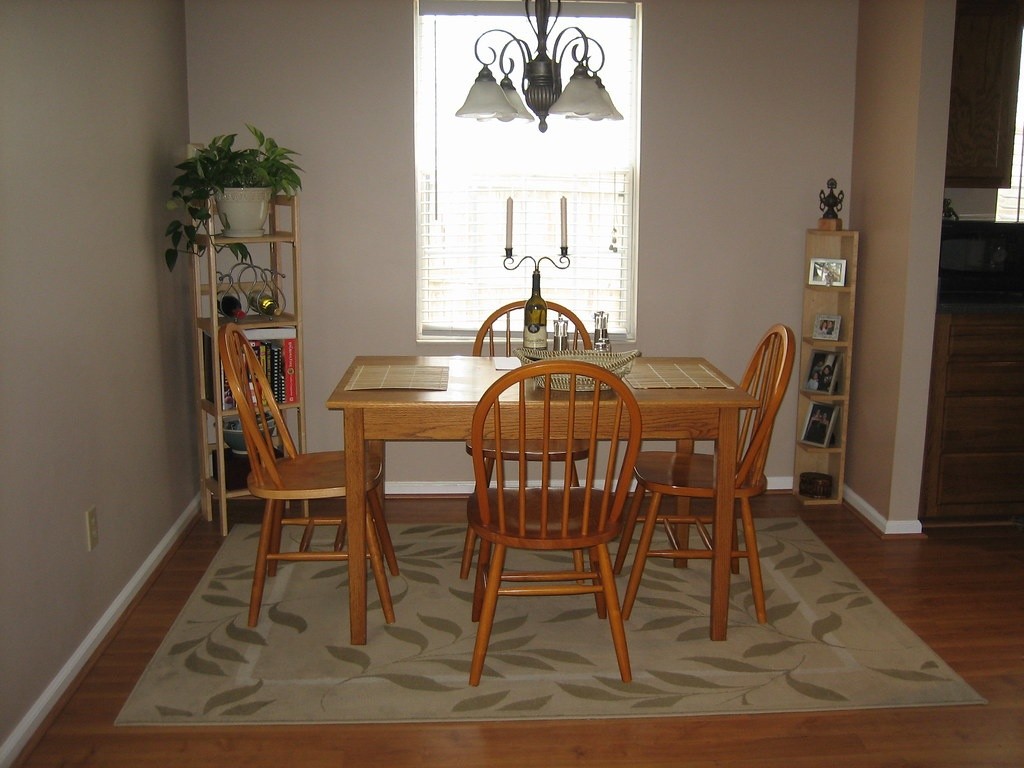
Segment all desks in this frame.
[323,356,760,636]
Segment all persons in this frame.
[820,321,834,335]
[812,408,830,425]
[808,358,833,389]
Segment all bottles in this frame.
[248,290,282,317]
[217,290,245,320]
[553,311,611,353]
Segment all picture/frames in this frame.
[797,401,839,447]
[812,314,842,341]
[808,258,846,287]
[801,348,842,395]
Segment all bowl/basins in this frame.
[215,417,277,455]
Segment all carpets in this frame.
[113,524,986,729]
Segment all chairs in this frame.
[460,299,593,579]
[470,361,633,685]
[213,322,399,626]
[614,326,796,625]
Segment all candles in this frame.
[560,196,567,247]
[506,197,513,250]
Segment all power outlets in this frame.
[86,505,99,551]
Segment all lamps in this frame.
[456,0,624,133]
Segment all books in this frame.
[205,327,297,410]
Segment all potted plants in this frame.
[164,122,306,270]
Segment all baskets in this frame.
[513,348,638,391]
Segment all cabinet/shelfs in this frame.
[944,0,1024,187]
[918,311,1024,527]
[793,229,859,505]
[189,188,309,537]
